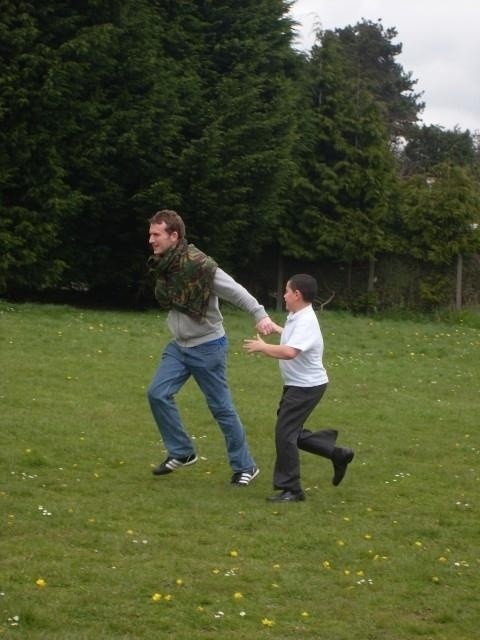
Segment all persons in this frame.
[146,209,274,487]
[243,274,354,501]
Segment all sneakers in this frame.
[230,465,259,486]
[152,452,198,475]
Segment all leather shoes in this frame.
[331,448,354,486]
[265,488,305,501]
